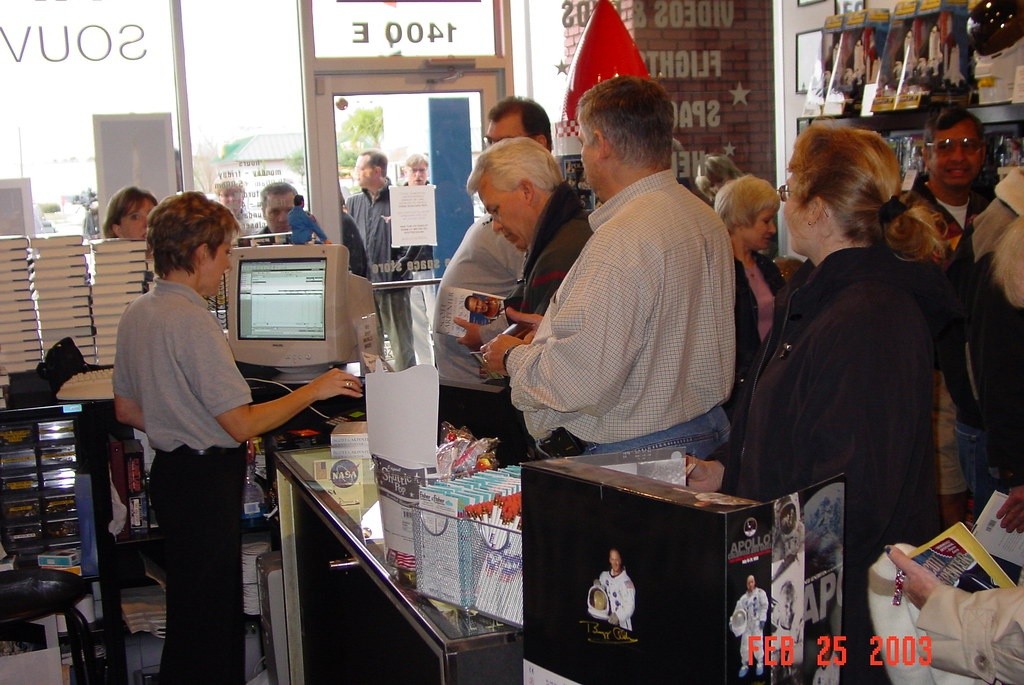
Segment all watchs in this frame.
[503,344,520,374]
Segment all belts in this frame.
[155,447,237,455]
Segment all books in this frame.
[907,521,1016,590]
[971,490,1024,566]
[434,287,507,338]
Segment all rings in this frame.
[346,381,352,387]
[482,353,488,363]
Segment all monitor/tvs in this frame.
[227,244,376,384]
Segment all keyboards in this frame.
[56,368,115,400]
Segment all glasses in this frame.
[927,136,981,155]
[780,183,829,218]
[482,137,495,148]
[407,167,426,174]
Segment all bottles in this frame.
[241,472,267,529]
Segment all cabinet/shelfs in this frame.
[0,401,101,580]
[269,447,525,685]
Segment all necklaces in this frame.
[745,268,754,277]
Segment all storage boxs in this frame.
[330,422,368,448]
[123,439,146,497]
[41,565,83,577]
[37,549,82,566]
[330,447,372,461]
[519,445,844,685]
[0,555,17,573]
[128,492,149,530]
[410,502,523,629]
[799,0,974,116]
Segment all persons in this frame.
[694,152,787,399]
[593,550,635,630]
[480,76,734,451]
[33,187,158,241]
[686,125,951,605]
[288,195,331,245]
[109,192,364,685]
[729,496,839,684]
[904,105,992,524]
[342,148,437,369]
[885,171,1024,685]
[214,175,297,246]
[432,95,595,467]
[465,295,505,322]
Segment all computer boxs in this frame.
[256,550,291,685]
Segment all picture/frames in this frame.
[794,28,824,94]
[834,0,866,15]
[797,116,809,137]
[796,0,826,8]
